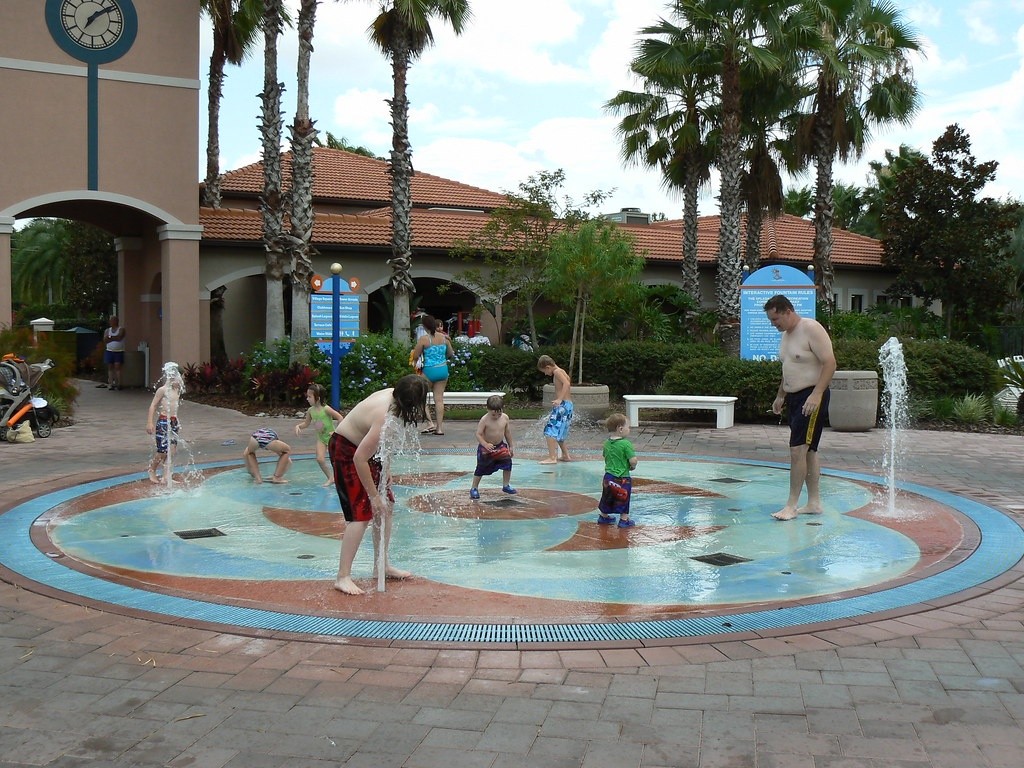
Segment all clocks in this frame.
[60,0,124,50]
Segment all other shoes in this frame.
[597,514,616,523]
[618,517,635,527]
[502,485,516,494]
[470,488,480,499]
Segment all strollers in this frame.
[0,353,61,441]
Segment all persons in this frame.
[328,374,429,595]
[243,427,292,484]
[469,394,518,499]
[597,413,638,528]
[412,309,454,436]
[102,316,126,392]
[764,294,837,521]
[295,384,344,488]
[511,330,534,353]
[145,371,182,485]
[536,353,574,465]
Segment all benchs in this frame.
[623,395,738,429]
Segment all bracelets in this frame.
[274,473,282,477]
[109,335,112,340]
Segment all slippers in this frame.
[421,427,437,433]
[432,432,444,435]
